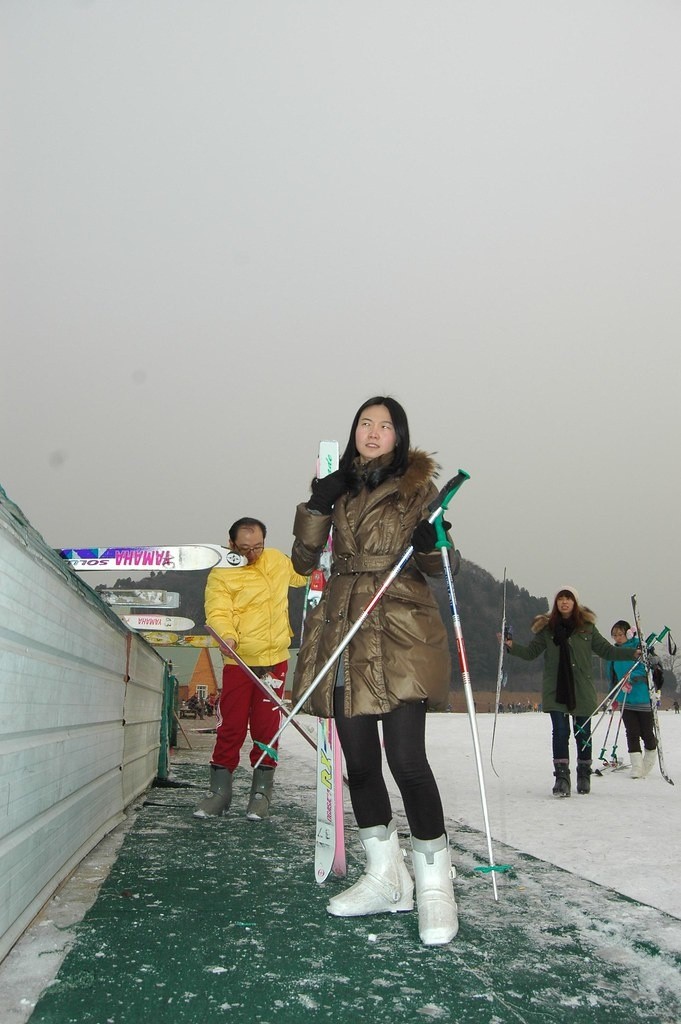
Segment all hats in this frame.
[550,586,579,606]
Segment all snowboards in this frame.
[205,625,351,789]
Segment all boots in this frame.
[409,831,461,948]
[193,765,232,818]
[243,766,276,818]
[627,751,647,780]
[642,748,657,777]
[550,759,572,799]
[576,759,594,794]
[330,816,414,916]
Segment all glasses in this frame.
[231,541,265,557]
[611,633,624,638]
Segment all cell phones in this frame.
[319,440,339,478]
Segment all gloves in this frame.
[411,518,453,555]
[307,464,354,514]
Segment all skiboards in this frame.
[490,566,674,786]
[51,544,250,647]
[306,440,348,884]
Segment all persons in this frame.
[495,589,642,798]
[191,516,312,821]
[186,691,220,719]
[445,699,614,714]
[672,698,680,714]
[606,620,664,780]
[289,397,461,946]
